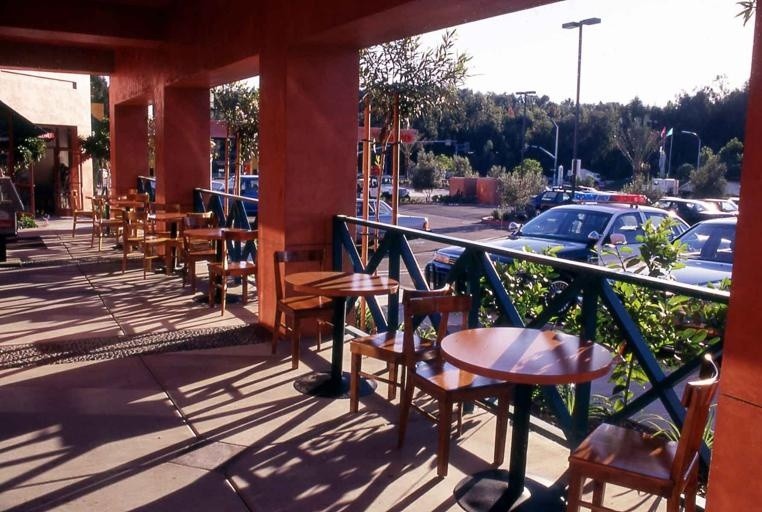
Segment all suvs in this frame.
[358,174,412,200]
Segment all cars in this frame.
[653,198,721,228]
[616,217,739,306]
[355,197,431,252]
[212,172,259,217]
[425,204,698,306]
[702,198,739,214]
[524,188,653,217]
[729,197,741,206]
[613,212,738,368]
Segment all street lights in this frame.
[513,89,537,168]
[558,14,606,200]
[681,126,705,174]
[542,112,562,192]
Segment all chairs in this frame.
[70,189,258,317]
